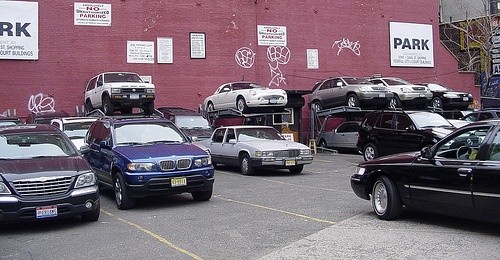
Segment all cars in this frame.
[0,117,23,129]
[317,121,365,155]
[365,74,434,110]
[349,117,500,220]
[0,124,102,221]
[49,116,101,152]
[445,107,500,130]
[203,81,288,114]
[415,83,475,110]
[193,125,314,176]
[307,76,395,111]
[157,106,215,143]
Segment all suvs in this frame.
[26,110,70,127]
[79,114,216,210]
[84,72,156,114]
[355,108,459,162]
